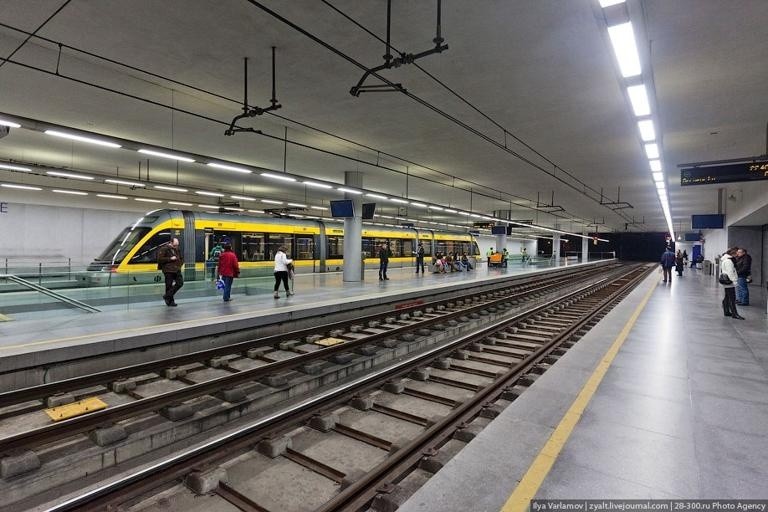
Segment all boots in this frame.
[286,289,294,296]
[384,275,389,280]
[273,290,280,298]
[379,275,383,280]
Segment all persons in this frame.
[682,249,689,266]
[553,250,556,259]
[732,246,740,301]
[431,250,474,273]
[520,247,528,263]
[688,253,704,269]
[217,244,241,304]
[157,237,186,308]
[486,246,495,267]
[734,248,753,305]
[377,242,391,281]
[209,242,225,281]
[719,246,745,320]
[660,246,676,283]
[501,249,508,268]
[675,248,685,276]
[413,242,425,274]
[273,245,294,299]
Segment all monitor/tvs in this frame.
[507,227,512,235]
[330,199,355,218]
[362,202,376,220]
[491,226,506,235]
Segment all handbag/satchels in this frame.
[287,264,296,278]
[158,249,166,269]
[742,276,753,282]
[718,274,732,285]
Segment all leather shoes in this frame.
[224,299,230,301]
[724,312,744,319]
[163,295,177,306]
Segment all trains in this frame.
[74,207,482,287]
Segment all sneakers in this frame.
[736,300,749,306]
